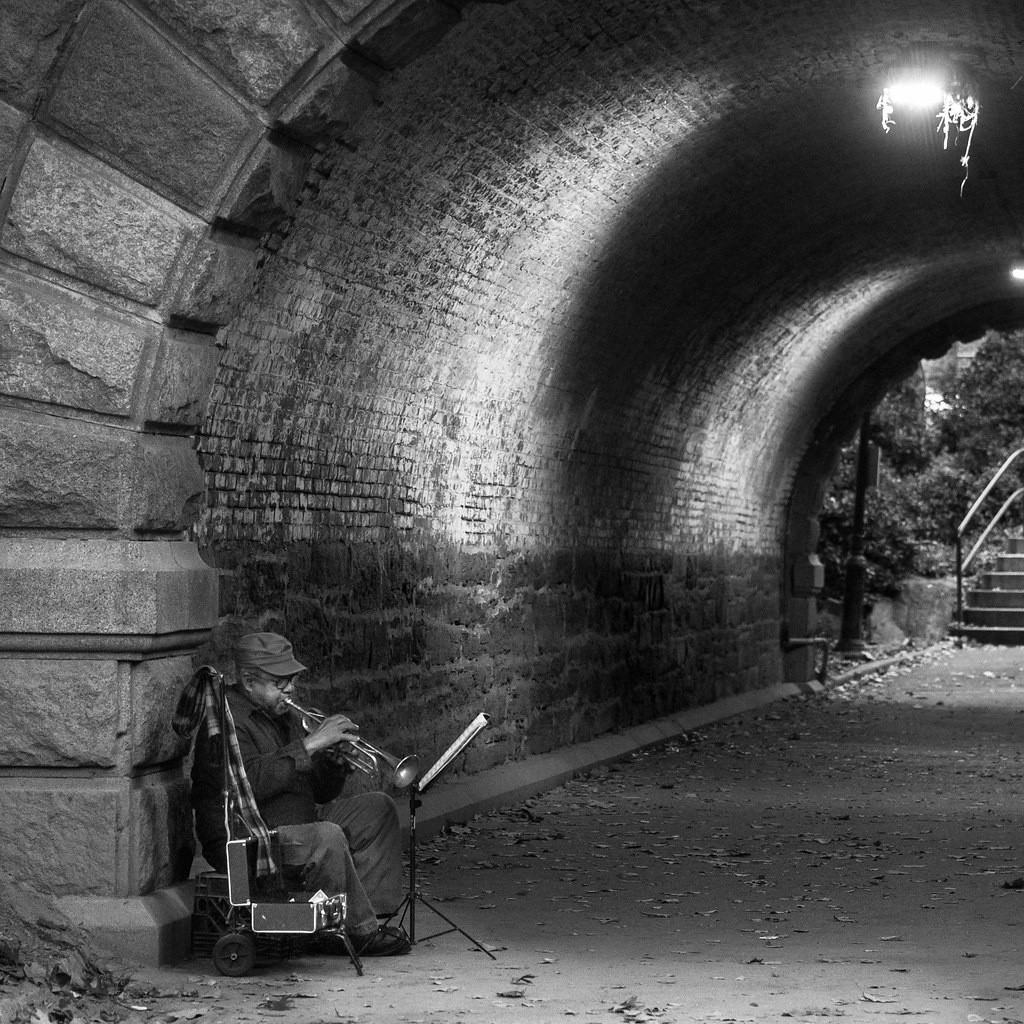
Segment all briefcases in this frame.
[226,829,347,933]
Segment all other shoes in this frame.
[340,925,407,956]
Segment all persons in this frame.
[191,633,412,956]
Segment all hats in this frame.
[232,632,307,676]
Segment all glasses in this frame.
[247,672,302,689]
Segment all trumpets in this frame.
[282,696,420,788]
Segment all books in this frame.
[419,712,492,793]
